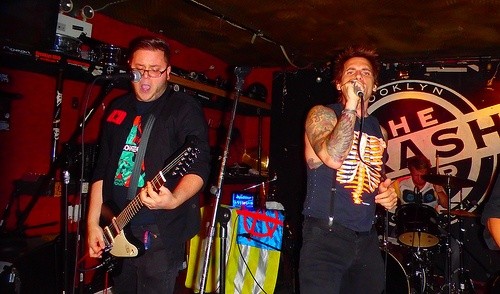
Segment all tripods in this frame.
[438,219,479,294]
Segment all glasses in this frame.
[127,61,170,78]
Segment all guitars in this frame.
[98,141,201,276]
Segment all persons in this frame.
[482,174,500,247]
[300,48,398,294]
[398,155,460,289]
[88,38,217,294]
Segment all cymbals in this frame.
[421,174,479,187]
[441,208,477,217]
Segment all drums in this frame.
[395,203,441,247]
[379,245,426,294]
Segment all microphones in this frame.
[354,87,364,97]
[100,69,142,83]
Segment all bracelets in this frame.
[343,109,357,113]
